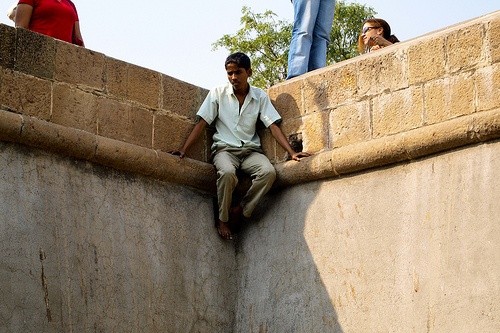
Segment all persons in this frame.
[13,0,84,48]
[167,53,311,241]
[356,20,399,55]
[285,0,335,79]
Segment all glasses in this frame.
[363,26,380,34]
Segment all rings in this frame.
[376,41,379,44]
